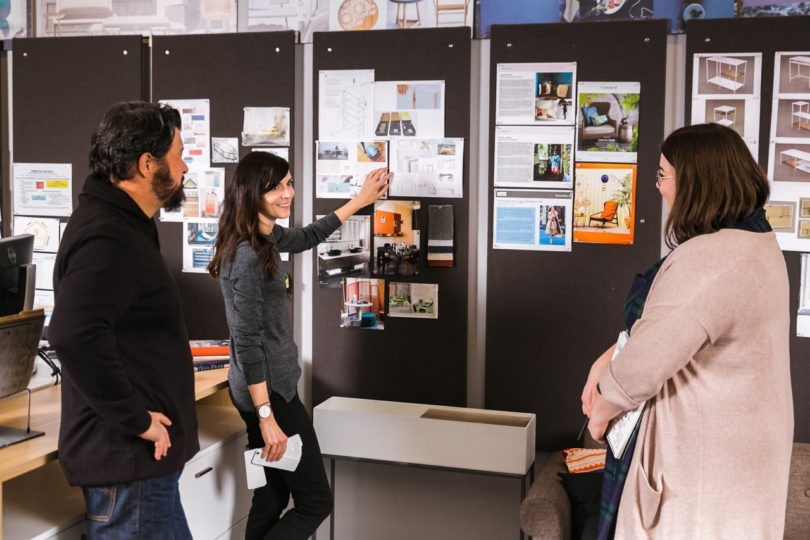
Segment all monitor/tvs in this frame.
[0,233,37,318]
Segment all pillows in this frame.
[559,447,607,473]
[556,469,603,540]
[580,105,598,125]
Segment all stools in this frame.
[361,313,377,327]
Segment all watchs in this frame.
[257,403,272,418]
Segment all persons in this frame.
[549,149,561,176]
[545,206,562,244]
[47,102,200,540]
[208,151,394,540]
[580,122,796,540]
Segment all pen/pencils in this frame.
[577,418,589,440]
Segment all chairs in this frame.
[588,200,619,229]
[581,102,618,150]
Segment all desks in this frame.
[377,246,420,275]
[780,148,810,172]
[573,213,587,229]
[313,395,537,540]
[345,301,373,327]
[789,56,810,87]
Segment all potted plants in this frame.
[611,172,633,230]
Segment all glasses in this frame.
[656,170,677,185]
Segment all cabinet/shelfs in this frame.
[0,368,255,540]
[792,101,810,130]
[375,210,402,235]
[705,57,748,94]
[714,105,737,128]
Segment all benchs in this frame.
[519,442,810,540]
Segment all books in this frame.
[189,340,231,372]
[604,330,650,461]
